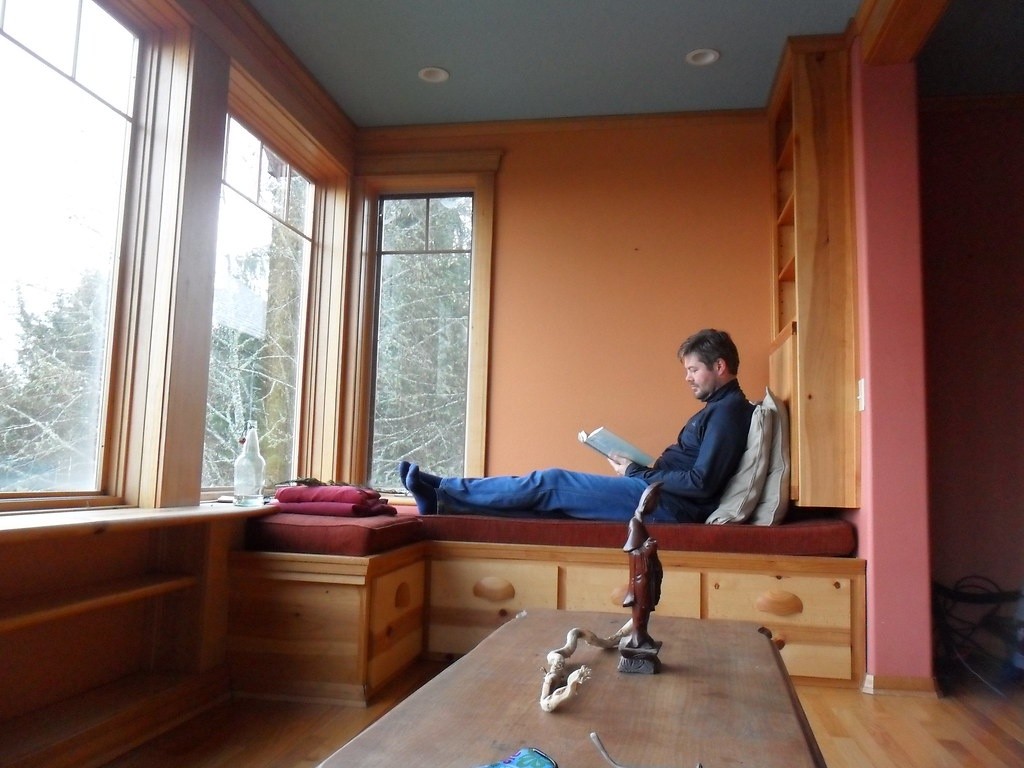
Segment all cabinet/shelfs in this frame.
[559,547,703,619]
[0,507,276,768]
[763,32,860,509]
[426,539,560,658]
[704,554,868,691]
[226,540,427,709]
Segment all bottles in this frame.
[233,420,265,506]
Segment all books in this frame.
[578,427,654,467]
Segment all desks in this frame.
[313,605,828,768]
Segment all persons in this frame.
[622,480,664,648]
[399,329,754,525]
